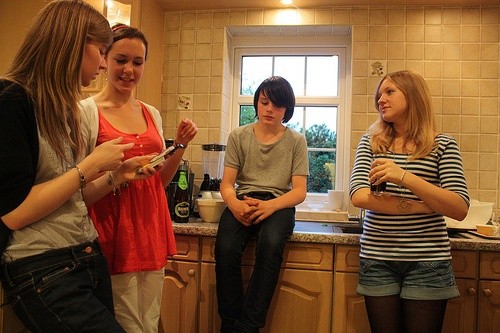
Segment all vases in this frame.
[328,189,344,211]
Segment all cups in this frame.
[348,200,361,221]
[327,189,345,211]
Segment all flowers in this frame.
[325,162,336,190]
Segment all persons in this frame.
[205,76,310,333]
[349,69,471,333]
[1,0,165,333]
[74,23,198,333]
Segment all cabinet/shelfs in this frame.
[159,230,500,333]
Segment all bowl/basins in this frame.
[195,198,228,223]
[443,202,496,229]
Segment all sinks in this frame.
[341,227,364,234]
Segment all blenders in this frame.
[199,145,226,198]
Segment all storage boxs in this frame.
[444,201,494,230]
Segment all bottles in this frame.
[174,170,190,224]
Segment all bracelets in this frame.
[107,170,121,196]
[174,139,187,149]
[399,170,407,188]
[70,165,86,190]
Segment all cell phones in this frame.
[138,146,175,172]
